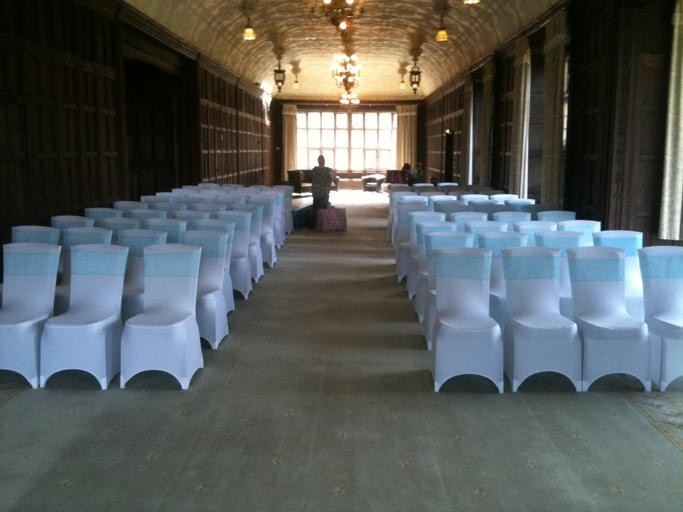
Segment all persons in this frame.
[310,155,339,231]
[390,163,415,184]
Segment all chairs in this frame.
[385,162,411,182]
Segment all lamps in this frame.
[408,48,421,93]
[290,67,300,89]
[238,0,257,40]
[435,5,449,42]
[272,56,285,90]
[397,68,406,90]
[328,5,365,105]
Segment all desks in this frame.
[361,172,384,192]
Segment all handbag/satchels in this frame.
[315,208,347,232]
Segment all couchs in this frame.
[285,167,339,195]
